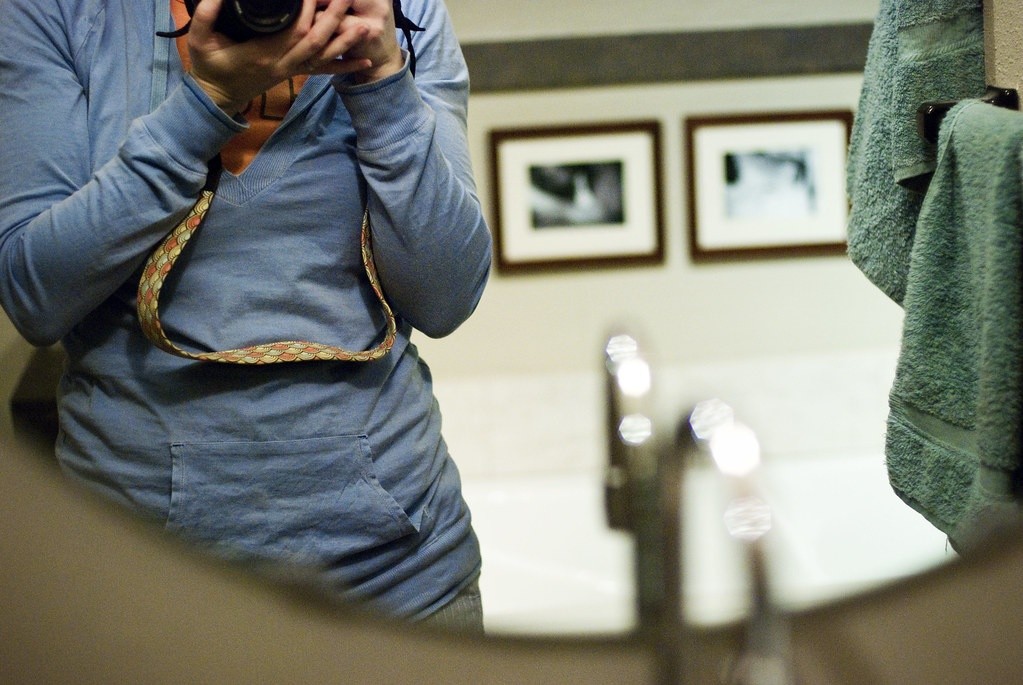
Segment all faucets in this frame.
[603,333,762,631]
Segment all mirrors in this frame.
[0,0,984,642]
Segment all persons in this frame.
[0,0,490,630]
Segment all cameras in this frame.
[184,0,329,43]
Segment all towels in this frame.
[885,98,1023,556]
[844,1,984,311]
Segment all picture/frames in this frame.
[684,111,856,263]
[488,120,664,273]
